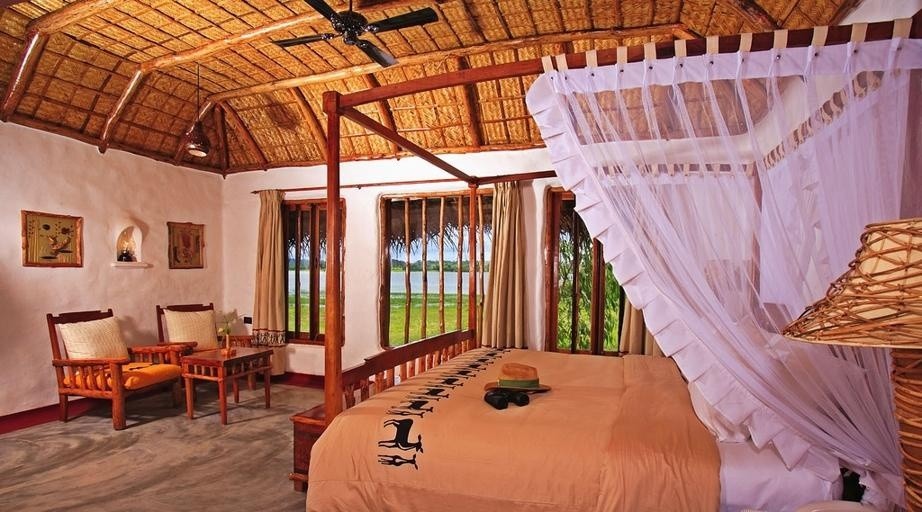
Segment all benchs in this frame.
[289,376,411,492]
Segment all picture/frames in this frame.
[21,211,83,268]
[167,222,205,269]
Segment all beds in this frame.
[306,9,922,512]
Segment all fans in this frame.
[269,0,438,68]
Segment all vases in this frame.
[224,334,232,352]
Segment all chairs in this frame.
[47,308,190,430]
[149,302,258,409]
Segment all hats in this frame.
[484,363,552,393]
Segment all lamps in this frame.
[783,218,922,509]
[181,65,213,159]
[112,224,148,267]
